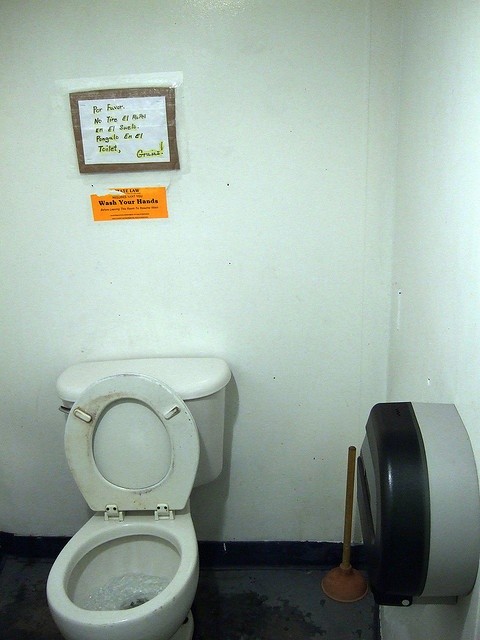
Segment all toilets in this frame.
[45,356,232,640]
